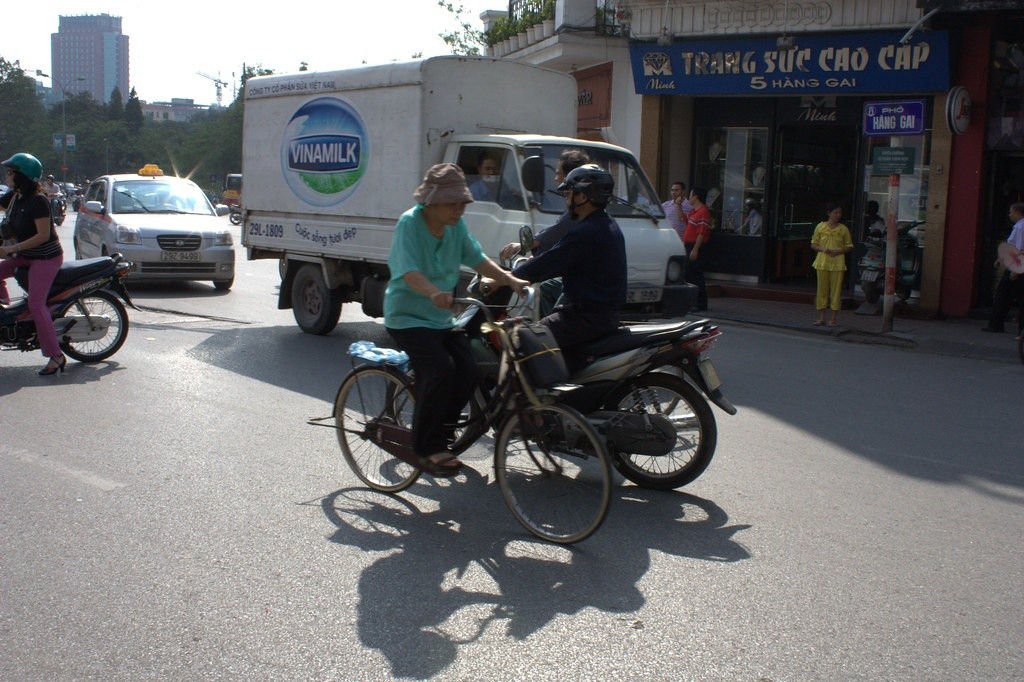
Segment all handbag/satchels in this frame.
[0,218,20,255]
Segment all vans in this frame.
[221,172,241,205]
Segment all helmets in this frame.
[1,152,43,186]
[557,162,615,209]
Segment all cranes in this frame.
[195,69,229,113]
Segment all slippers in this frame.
[827,321,840,327]
[813,319,827,325]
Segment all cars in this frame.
[71,162,234,291]
[0,180,82,212]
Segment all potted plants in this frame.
[481,0,555,56]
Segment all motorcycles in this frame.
[0,235,144,364]
[384,222,738,492]
[229,203,243,225]
[41,194,66,227]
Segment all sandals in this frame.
[418,450,465,472]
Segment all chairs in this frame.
[463,165,481,188]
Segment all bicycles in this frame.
[331,285,613,546]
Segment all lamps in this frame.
[656,0,674,46]
[775,0,794,50]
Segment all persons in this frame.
[383,163,530,467]
[500,148,590,260]
[980,203,1024,341]
[82,180,91,195]
[238,190,241,208]
[863,201,886,239]
[469,158,517,209]
[479,163,627,346]
[661,182,710,312]
[811,201,853,327]
[734,198,762,235]
[0,153,66,375]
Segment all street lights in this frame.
[35,68,86,186]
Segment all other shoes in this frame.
[691,305,708,312]
[981,323,1006,333]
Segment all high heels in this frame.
[38,353,66,375]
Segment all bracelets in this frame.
[16,244,20,252]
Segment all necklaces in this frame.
[710,145,721,160]
[708,190,719,203]
[755,168,764,181]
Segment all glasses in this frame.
[7,169,17,176]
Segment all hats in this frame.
[415,161,474,205]
[46,174,55,182]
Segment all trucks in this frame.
[237,53,700,336]
